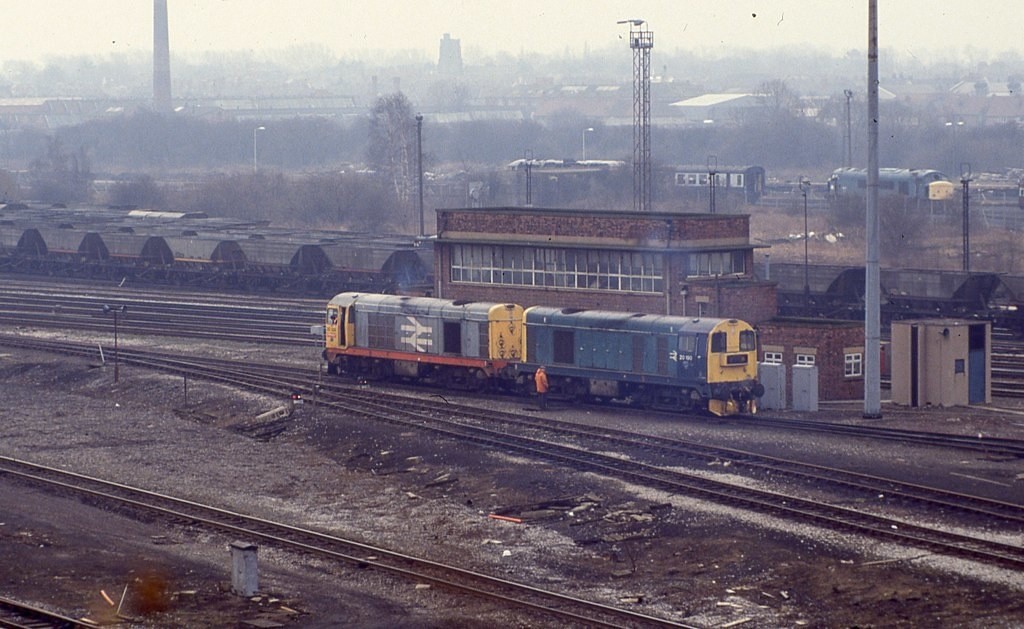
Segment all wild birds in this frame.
[776,11,785,26]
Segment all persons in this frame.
[535,366,548,410]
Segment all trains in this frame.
[831,167,956,209]
[507,157,766,204]
[320,288,766,419]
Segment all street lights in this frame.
[102,304,127,382]
[797,175,812,294]
[253,126,266,171]
[842,88,854,166]
[582,127,594,160]
